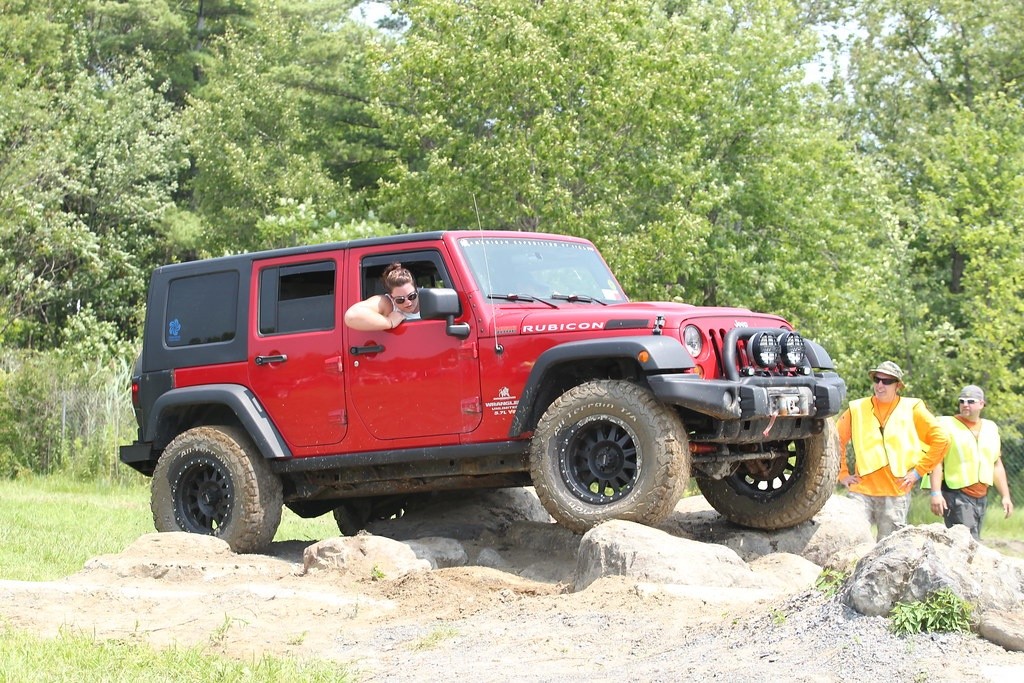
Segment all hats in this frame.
[868,361,905,385]
[958,384,983,402]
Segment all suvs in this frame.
[119,231,848,554]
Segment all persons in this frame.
[929,384,1013,544]
[343,261,426,331]
[837,360,950,542]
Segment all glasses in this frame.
[960,399,975,404]
[873,375,899,385]
[395,293,416,304]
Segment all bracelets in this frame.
[912,470,922,481]
[388,316,394,328]
[931,490,943,496]
